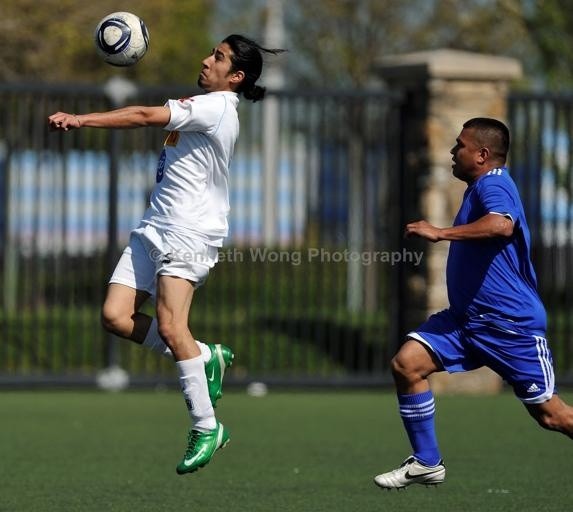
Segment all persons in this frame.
[48,35,291,475]
[373,118,572,491]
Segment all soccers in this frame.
[95,11,149,66]
[249,381,267,398]
[97,366,129,392]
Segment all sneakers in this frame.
[374,456,445,488]
[176,421,230,474]
[204,343,234,408]
